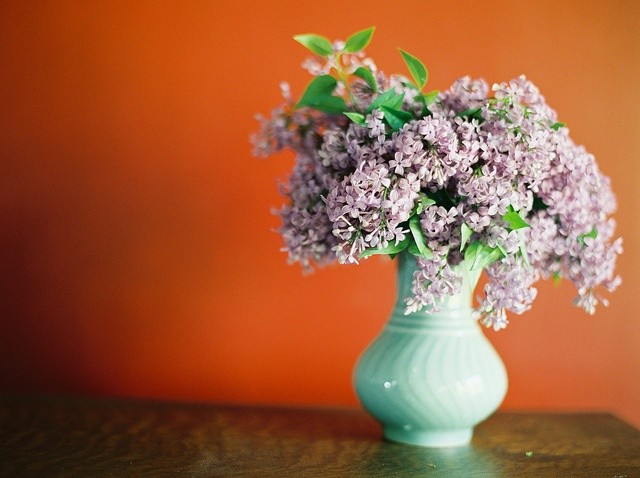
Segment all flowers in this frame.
[250,26,623,330]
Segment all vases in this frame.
[353,248,509,449]
